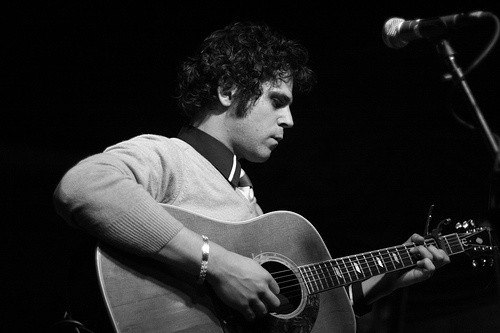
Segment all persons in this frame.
[49,17,450,333]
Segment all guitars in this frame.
[88,205,494,332]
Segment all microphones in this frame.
[382,10,491,50]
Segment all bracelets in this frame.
[194,234,211,286]
